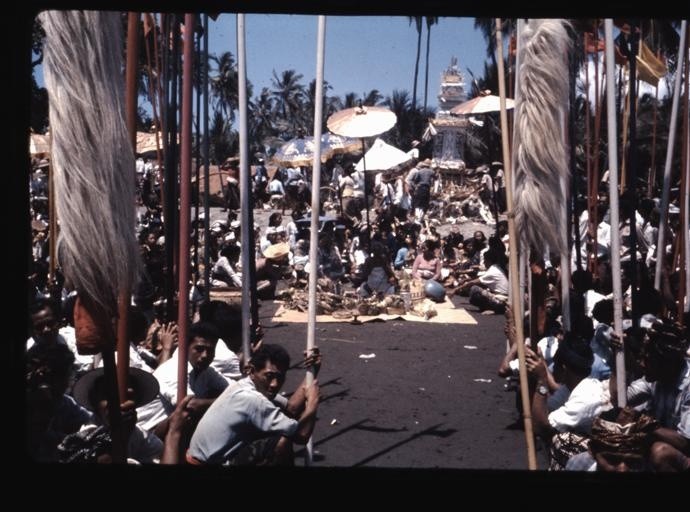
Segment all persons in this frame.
[322,153,517,320]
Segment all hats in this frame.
[264,243,290,259]
[72,366,159,412]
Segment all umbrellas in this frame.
[353,134,415,200]
[30,131,52,158]
[325,99,400,248]
[448,88,517,166]
[133,126,184,182]
[271,131,362,200]
